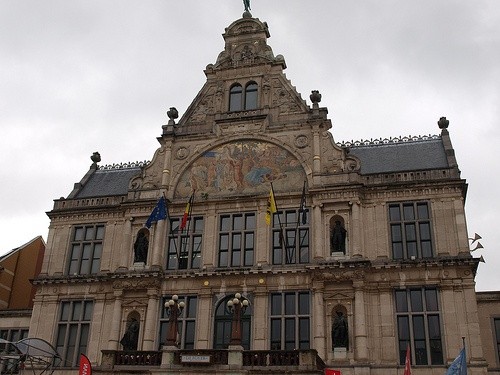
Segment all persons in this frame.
[134,231,149,262]
[332,219,347,252]
[124,317,139,349]
[332,310,348,346]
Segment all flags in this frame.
[180,194,192,231]
[265,186,276,224]
[445,346,467,375]
[143,193,167,230]
[300,184,307,225]
[404,343,411,375]
